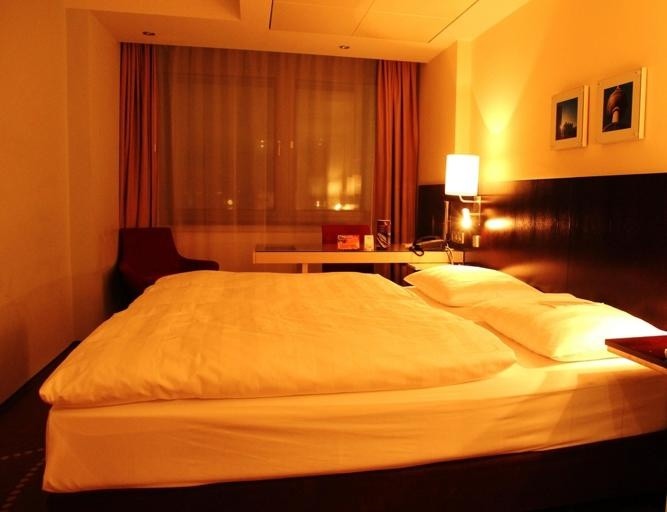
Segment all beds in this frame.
[37,172,666,511]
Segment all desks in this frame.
[252,241,464,273]
[604,335,666,375]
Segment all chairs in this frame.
[117,226,219,294]
[321,223,375,274]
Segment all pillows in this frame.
[475,291,666,363]
[403,263,544,308]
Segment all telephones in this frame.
[412,235,447,252]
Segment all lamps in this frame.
[445,154,481,225]
